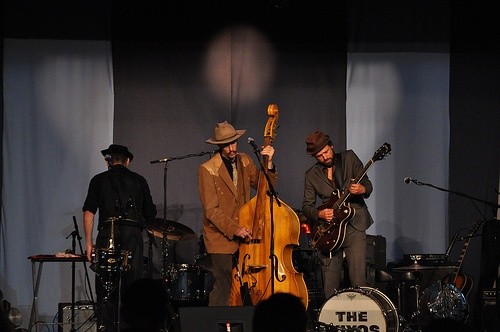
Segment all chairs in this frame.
[375,224,474,332]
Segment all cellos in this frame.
[228,104,308,310]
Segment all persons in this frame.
[200,122,278,307]
[84,143,156,331]
[303,131,374,299]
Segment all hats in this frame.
[305,129,331,155]
[101,144,133,162]
[205,121,247,144]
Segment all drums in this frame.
[317,284,400,332]
[418,283,472,332]
[94,245,213,311]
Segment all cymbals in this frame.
[143,217,196,241]
[106,217,137,222]
[393,264,459,271]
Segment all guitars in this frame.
[310,142,392,258]
[442,220,484,299]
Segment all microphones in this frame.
[403,177,420,184]
[248,137,261,153]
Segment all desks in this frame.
[27,253,84,332]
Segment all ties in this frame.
[231,162,238,190]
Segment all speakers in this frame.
[168,306,254,332]
[480,288,497,332]
[366,234,387,272]
